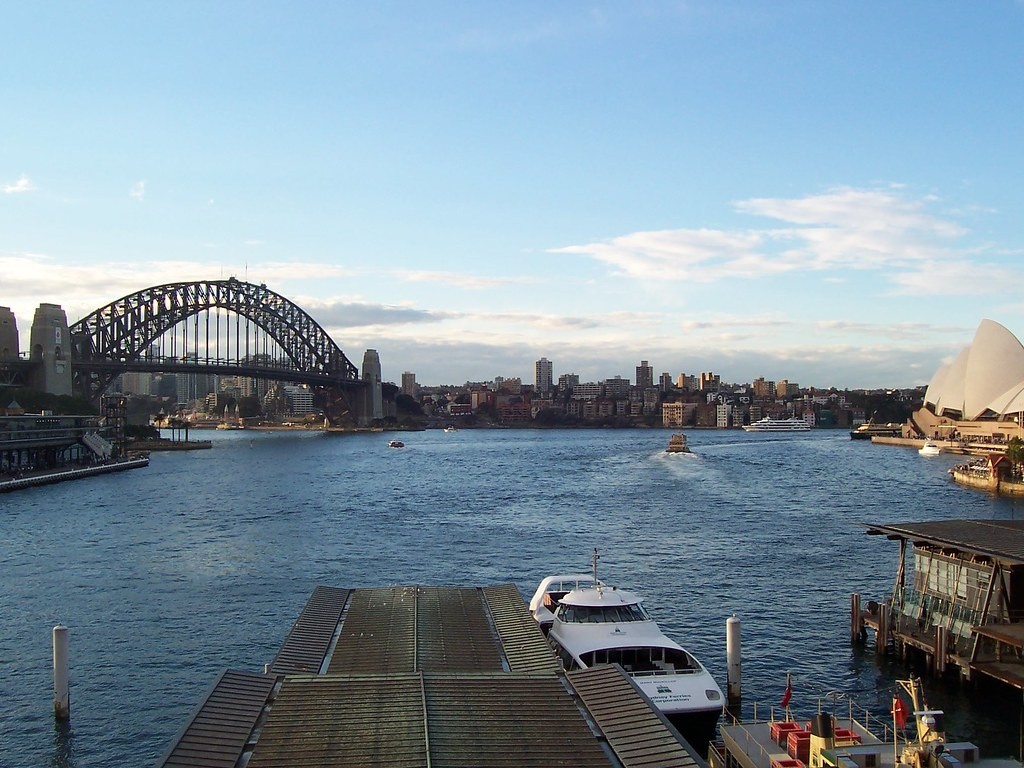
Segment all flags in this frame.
[895,699,908,728]
[780,686,792,707]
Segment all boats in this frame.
[918,442,941,456]
[387,440,405,448]
[532,548,726,733]
[665,434,690,454]
[443,425,458,433]
[741,413,812,432]
